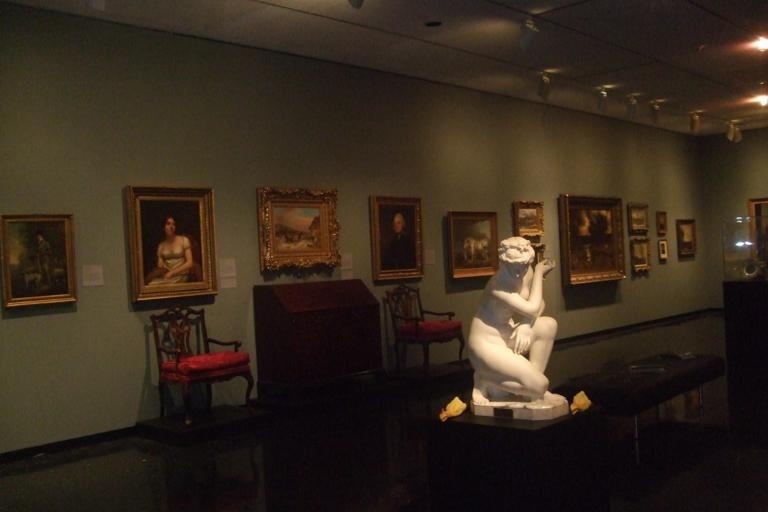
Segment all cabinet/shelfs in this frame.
[251,277,384,417]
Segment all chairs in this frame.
[147,306,257,423]
[381,286,466,380]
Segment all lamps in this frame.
[535,69,743,146]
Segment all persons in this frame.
[390,212,410,270]
[144,216,193,291]
[466,235,560,399]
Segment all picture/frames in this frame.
[366,193,429,282]
[627,204,701,274]
[557,190,629,288]
[121,183,219,306]
[254,184,342,276]
[511,197,545,245]
[441,208,503,281]
[0,211,78,311]
